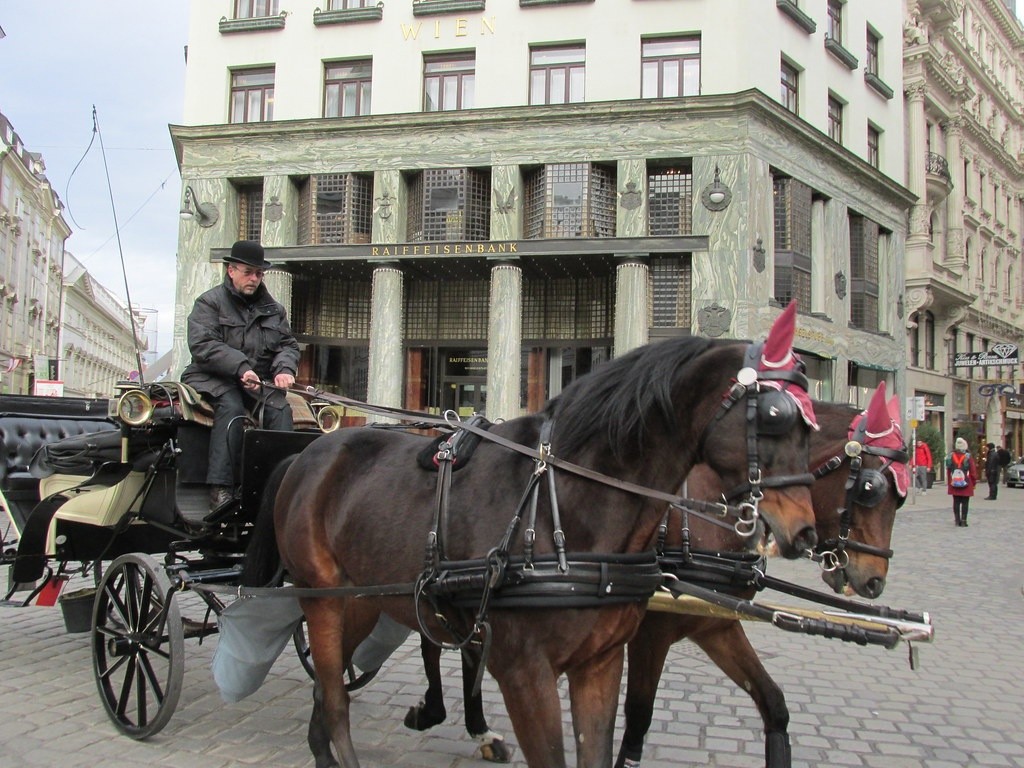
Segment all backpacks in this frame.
[945,452,970,488]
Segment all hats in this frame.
[955,438,969,450]
[223,240,272,269]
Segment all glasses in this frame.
[232,265,265,279]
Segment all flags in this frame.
[2,357,23,375]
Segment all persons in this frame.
[995,445,1007,484]
[181,240,300,515]
[985,443,1001,500]
[1010,449,1019,462]
[908,440,932,495]
[947,437,978,527]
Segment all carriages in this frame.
[0,302,938,767]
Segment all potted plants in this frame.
[908,420,946,489]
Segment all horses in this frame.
[241,302,817,768]
[402,380,911,768]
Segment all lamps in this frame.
[178,184,219,228]
[700,162,732,213]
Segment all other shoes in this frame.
[922,491,926,496]
[916,487,921,494]
[956,519,961,526]
[984,495,996,500]
[207,485,233,512]
[961,520,969,527]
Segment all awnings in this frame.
[155,372,172,382]
[133,348,172,382]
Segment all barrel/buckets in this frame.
[58,567,108,633]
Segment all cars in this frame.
[1007,456,1024,487]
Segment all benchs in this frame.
[0,395,121,484]
[130,384,314,484]
